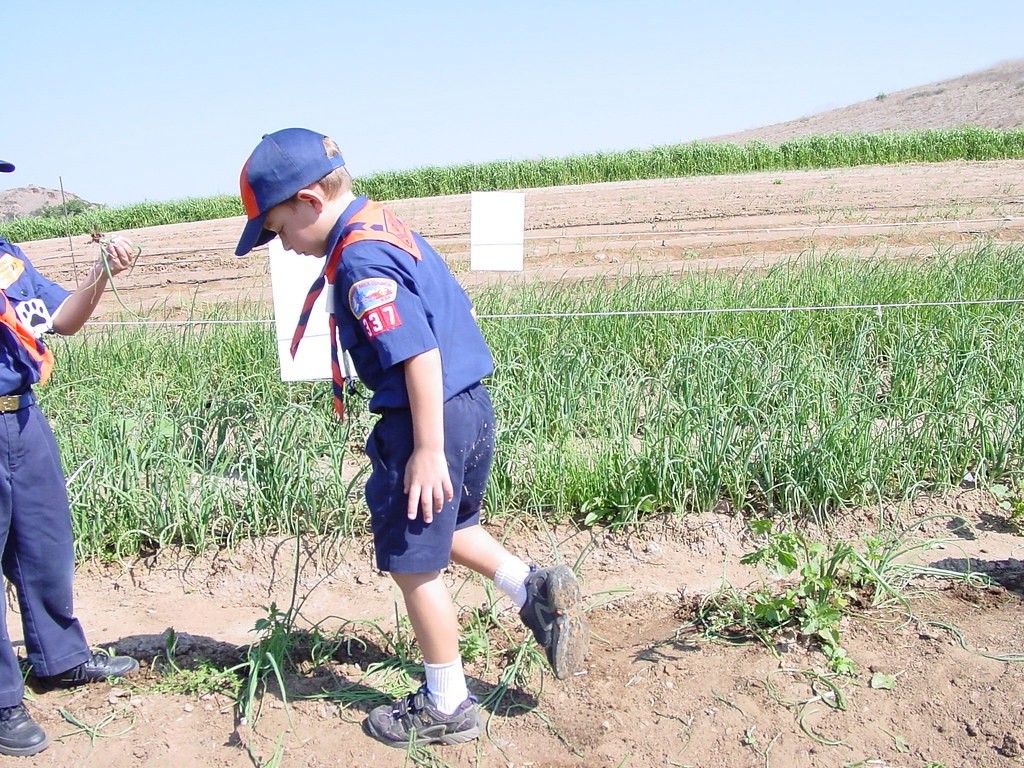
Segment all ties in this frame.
[290,200,422,423]
[0,289,54,386]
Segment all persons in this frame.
[235,127,588,750]
[1,159,140,760]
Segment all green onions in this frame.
[74,231,152,321]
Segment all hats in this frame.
[0,160,15,172]
[235,128,344,256]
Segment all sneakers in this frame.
[39,649,141,689]
[0,703,49,756]
[367,682,480,748]
[518,562,588,681]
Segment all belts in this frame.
[0,390,37,413]
